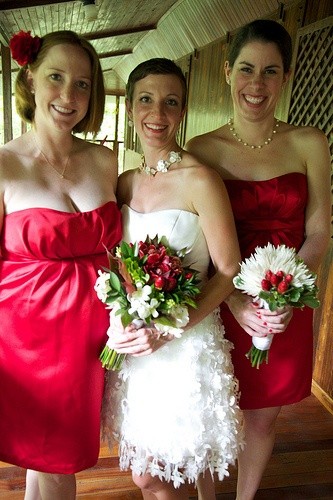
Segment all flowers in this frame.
[93,233,203,372]
[232,242,322,370]
[9,29,40,66]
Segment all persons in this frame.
[0,30,122,500]
[116,57,243,500]
[186,21,329,500]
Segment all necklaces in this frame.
[31,132,73,179]
[227,116,279,149]
[139,147,183,176]
